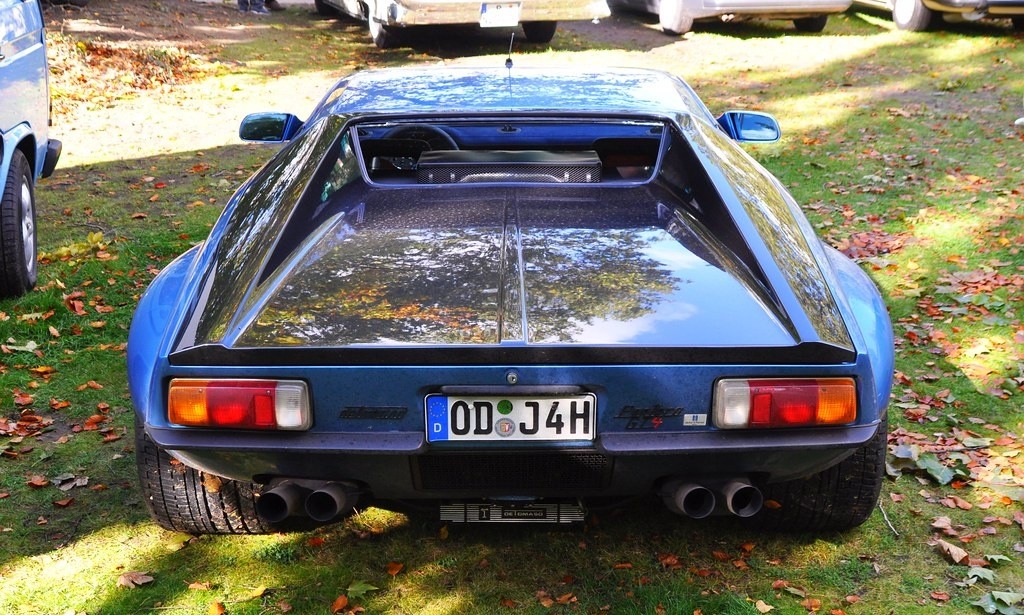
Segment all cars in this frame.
[124,58,901,541]
[857,0,1023,31]
[610,0,853,37]
[315,0,613,46]
[0,0,63,298]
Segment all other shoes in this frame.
[265,1,286,10]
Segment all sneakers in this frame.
[250,5,272,16]
[239,4,248,12]
